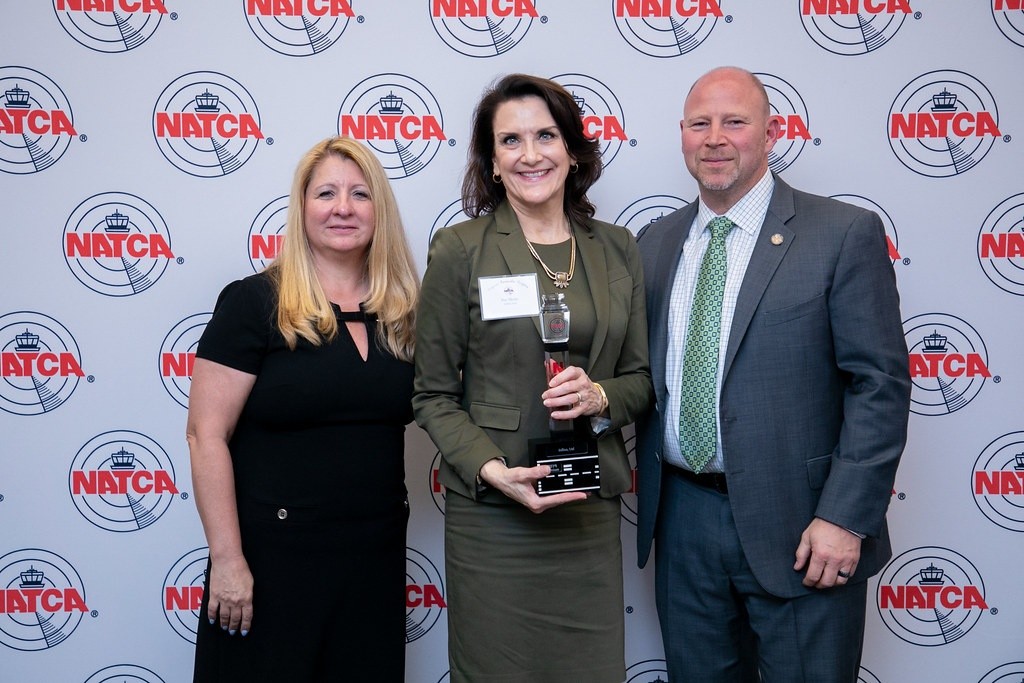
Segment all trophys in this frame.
[527,292,601,496]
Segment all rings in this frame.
[838,570,850,578]
[576,392,581,404]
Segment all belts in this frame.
[662,461,728,494]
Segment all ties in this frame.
[679,215,736,475]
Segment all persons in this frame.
[412,73,653,683]
[634,65,913,683]
[186,136,421,683]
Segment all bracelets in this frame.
[595,382,608,417]
[477,457,506,488]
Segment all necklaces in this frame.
[525,212,576,288]
